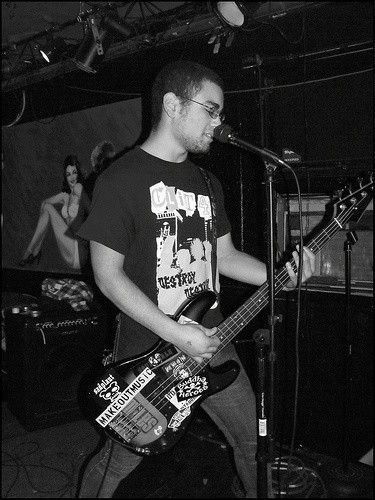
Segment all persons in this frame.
[75,59,316,499]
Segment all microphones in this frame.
[214,124,291,170]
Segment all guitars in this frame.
[80,172,375,456]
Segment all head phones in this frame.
[4,294,42,318]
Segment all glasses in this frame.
[175,94,226,123]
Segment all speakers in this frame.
[279,195,375,291]
[4,301,118,432]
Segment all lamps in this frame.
[212,1,268,30]
[74,21,115,77]
[100,9,135,44]
[39,33,67,64]
[2,61,29,75]
